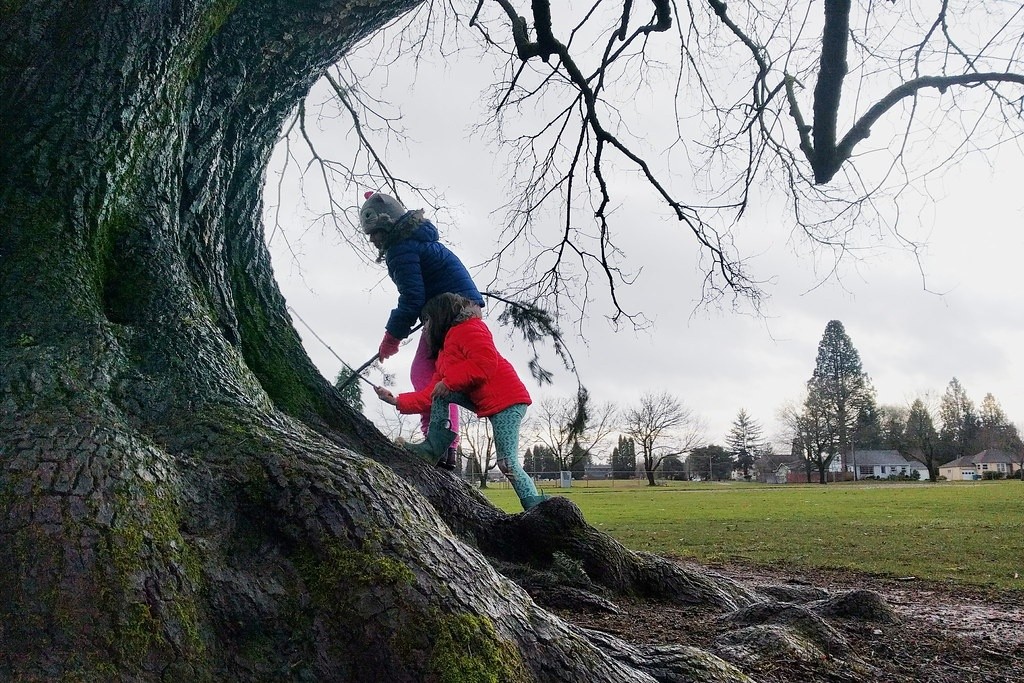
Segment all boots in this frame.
[520,489,548,511]
[403,418,458,467]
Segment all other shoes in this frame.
[437,447,457,471]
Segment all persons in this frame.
[360,191,486,470]
[373,290,545,509]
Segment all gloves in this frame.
[378,332,400,363]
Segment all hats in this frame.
[359,191,407,232]
